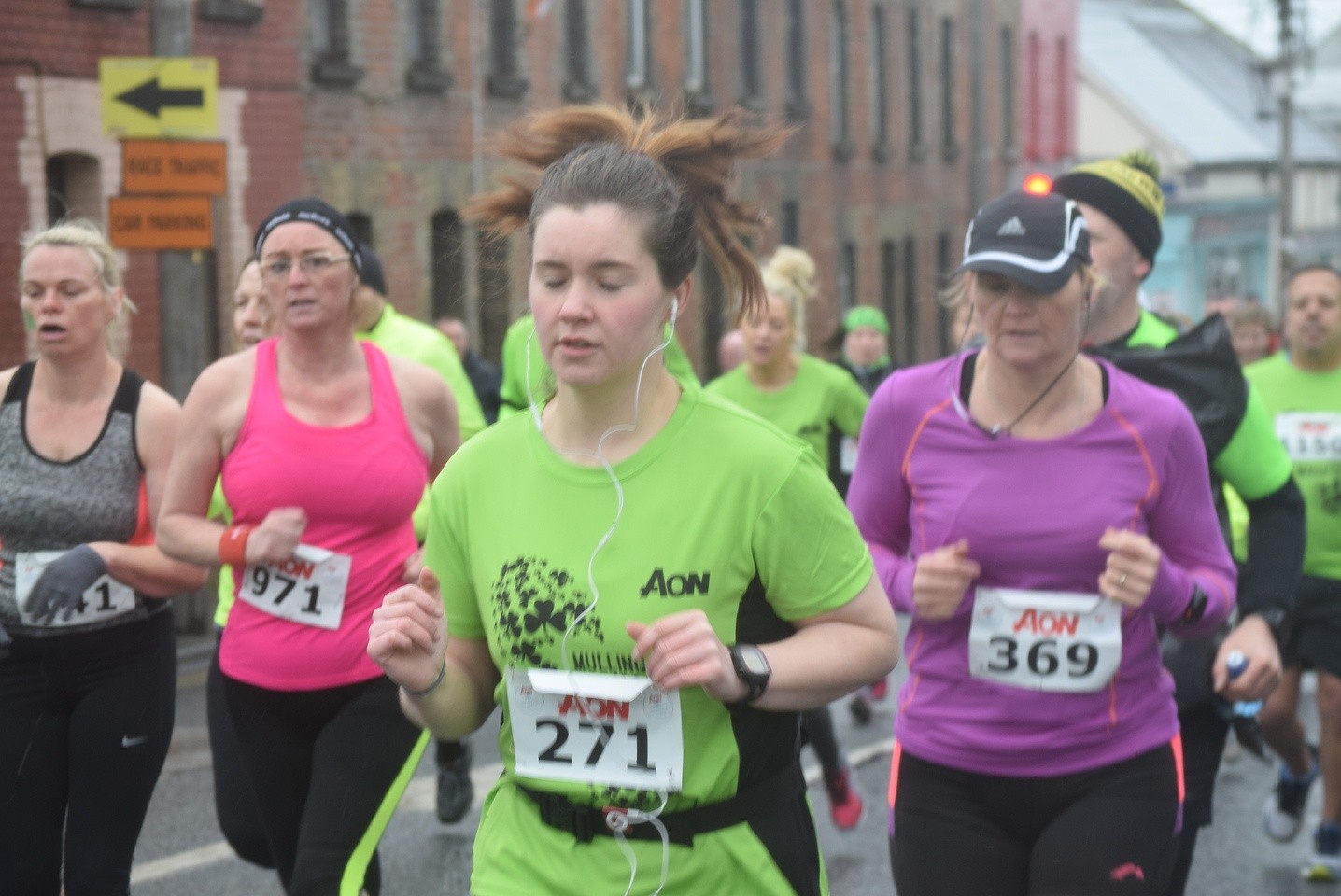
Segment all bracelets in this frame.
[218,524,252,564]
[408,658,446,697]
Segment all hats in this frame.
[254,193,386,291]
[946,182,1094,295]
[1052,147,1166,266]
[839,302,890,338]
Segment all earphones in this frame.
[1086,289,1091,302]
[669,295,679,325]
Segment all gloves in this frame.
[23,541,109,628]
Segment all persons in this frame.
[362,94,904,896]
[205,247,274,632]
[0,220,211,896]
[1042,144,1340,895]
[698,236,916,834]
[150,196,463,895]
[846,191,1240,895]
[343,239,486,816]
[495,312,697,420]
[436,318,500,422]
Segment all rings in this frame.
[1118,575,1130,585]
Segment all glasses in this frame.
[246,250,353,283]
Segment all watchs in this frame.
[723,643,775,714]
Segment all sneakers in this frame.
[1299,821,1341,885]
[827,762,865,831]
[1260,744,1318,843]
[429,737,475,822]
[871,676,889,702]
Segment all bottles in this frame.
[1230,655,1263,717]
